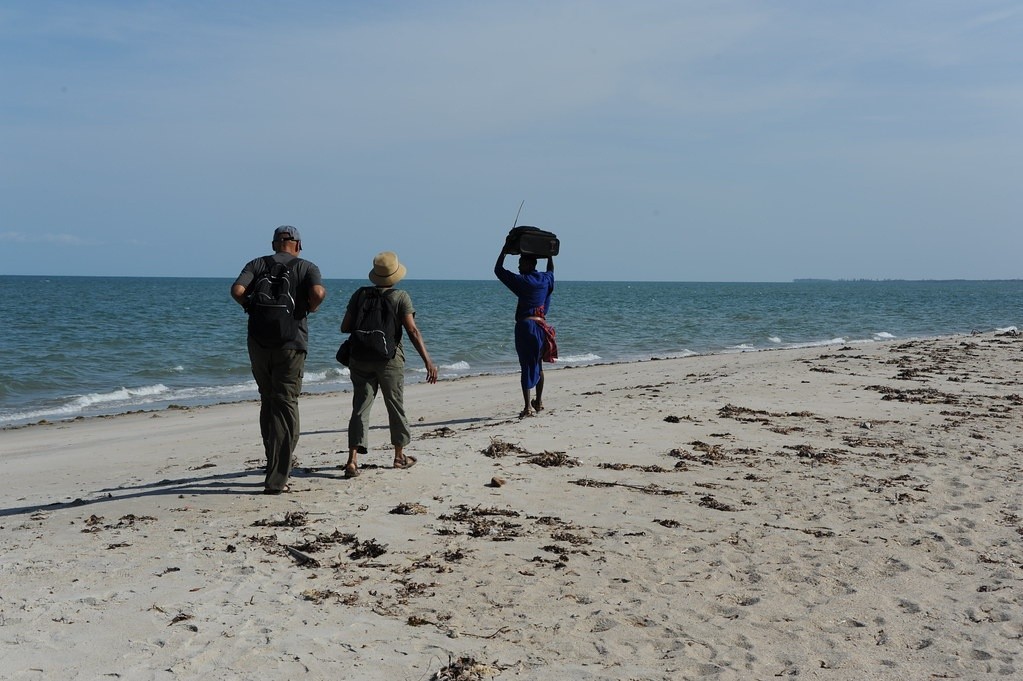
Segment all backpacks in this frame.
[245,256,310,350]
[350,287,402,370]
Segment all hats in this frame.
[274,226,302,250]
[369,252,406,286]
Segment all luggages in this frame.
[505,225,559,258]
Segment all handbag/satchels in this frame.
[336,340,351,367]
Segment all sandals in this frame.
[263,485,292,495]
[393,454,417,469]
[531,399,545,412]
[344,460,360,477]
[519,407,531,417]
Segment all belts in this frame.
[525,317,542,320]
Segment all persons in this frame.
[340,257,438,477]
[495,234,556,420]
[231,225,327,494]
